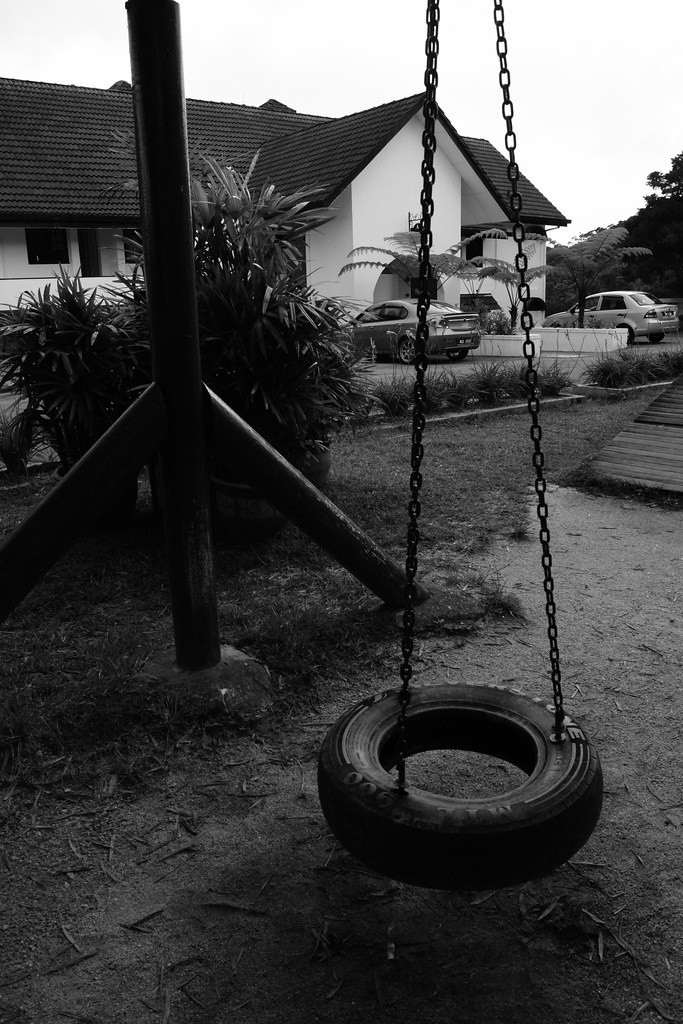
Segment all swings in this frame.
[311,1,601,891]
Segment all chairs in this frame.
[387,311,396,316]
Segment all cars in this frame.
[342,295,480,363]
[540,290,680,344]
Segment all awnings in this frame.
[460,296,502,312]
